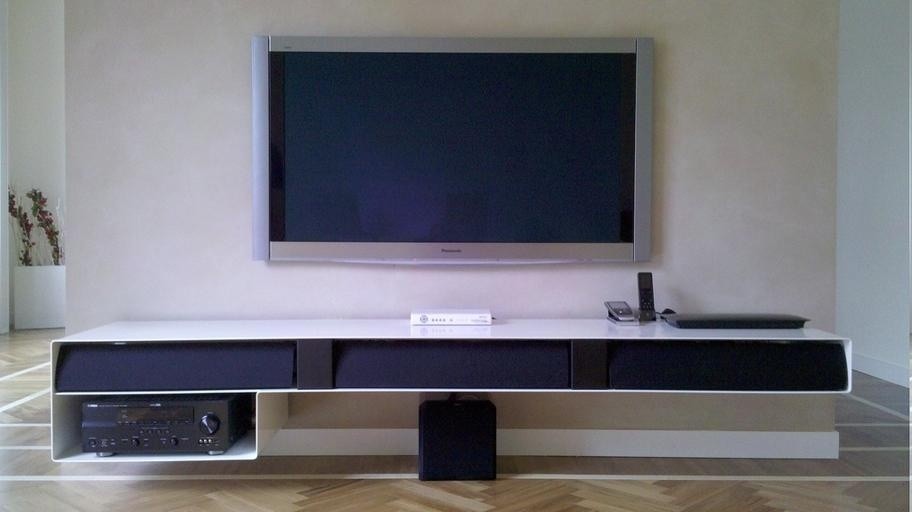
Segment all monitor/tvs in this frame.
[252,34,654,263]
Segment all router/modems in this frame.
[411,309,492,325]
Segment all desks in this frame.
[49,313,854,461]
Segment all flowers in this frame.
[8,179,64,266]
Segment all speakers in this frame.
[419,399,496,481]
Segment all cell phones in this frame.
[605,301,635,321]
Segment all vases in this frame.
[13,266,64,332]
